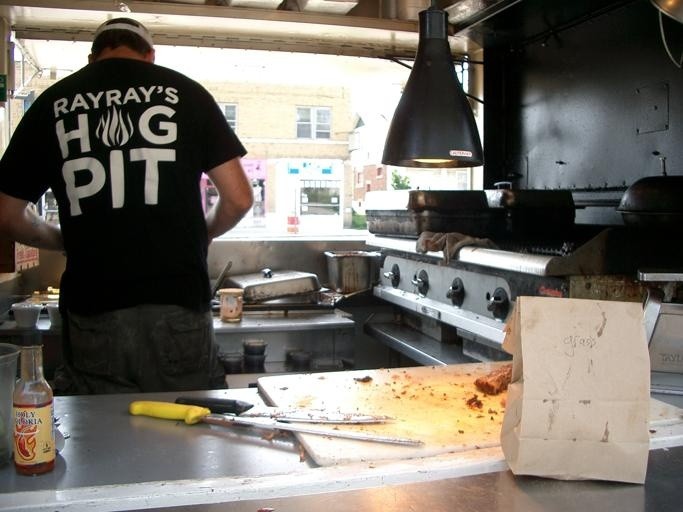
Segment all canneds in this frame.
[218,287,244,322]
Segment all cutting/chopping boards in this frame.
[255,356,683,468]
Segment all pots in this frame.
[616,156,683,250]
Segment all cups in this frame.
[12,302,43,330]
[45,301,64,327]
[0,341,22,467]
[217,286,244,323]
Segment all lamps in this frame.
[381,0,484,170]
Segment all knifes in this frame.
[128,400,425,447]
[173,395,396,429]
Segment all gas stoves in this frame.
[365,223,624,347]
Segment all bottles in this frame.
[11,328,56,476]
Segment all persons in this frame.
[0,16,253,397]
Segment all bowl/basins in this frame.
[220,340,268,372]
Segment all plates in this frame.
[25,297,59,305]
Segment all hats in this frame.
[93,18,154,47]
